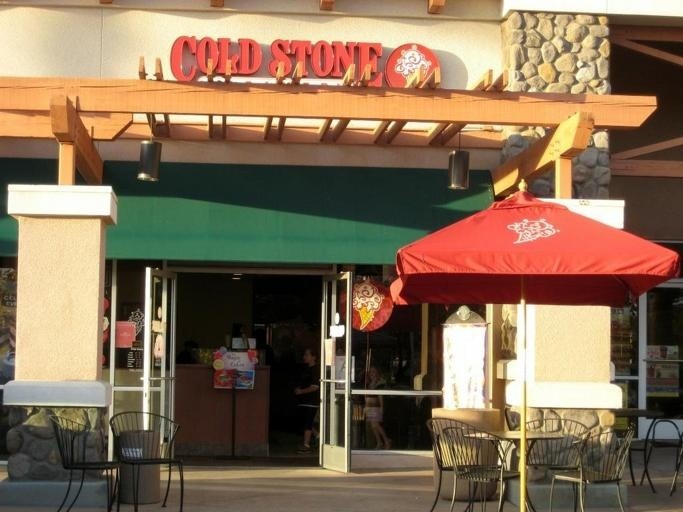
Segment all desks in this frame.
[618,406,683,496]
[465,431,564,511]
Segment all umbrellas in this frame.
[390,190,680,512]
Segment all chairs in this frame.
[108,411,184,511]
[526,418,590,511]
[448,427,538,510]
[549,430,635,510]
[50,417,124,511]
[427,415,504,511]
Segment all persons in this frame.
[293,348,320,452]
[362,366,392,450]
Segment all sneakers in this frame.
[295,445,314,455]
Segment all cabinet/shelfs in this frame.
[174,364,270,462]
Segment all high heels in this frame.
[374,442,384,450]
[385,439,394,449]
